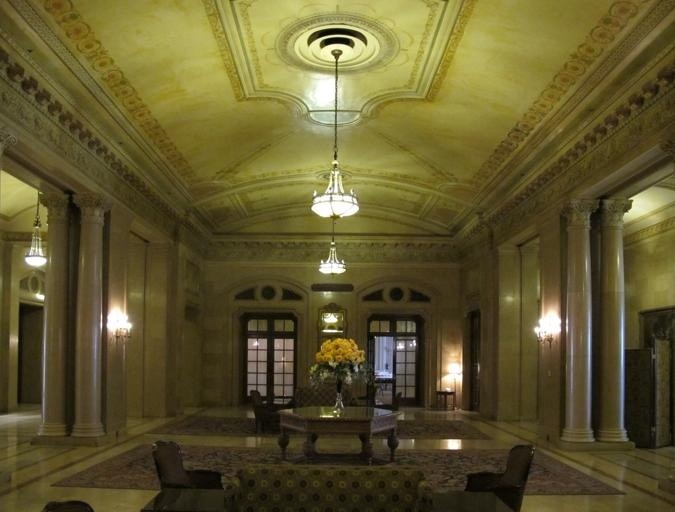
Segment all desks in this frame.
[433,390,456,411]
[276,405,403,467]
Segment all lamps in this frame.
[23,187,48,267]
[311,49,361,277]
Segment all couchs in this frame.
[286,383,361,407]
[224,462,436,511]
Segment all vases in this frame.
[333,380,345,412]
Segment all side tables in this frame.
[433,491,515,511]
[140,485,228,511]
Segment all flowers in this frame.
[307,338,376,394]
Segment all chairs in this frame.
[249,389,279,434]
[374,391,403,411]
[152,443,223,490]
[464,445,535,512]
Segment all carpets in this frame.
[144,411,491,441]
[50,441,627,494]
